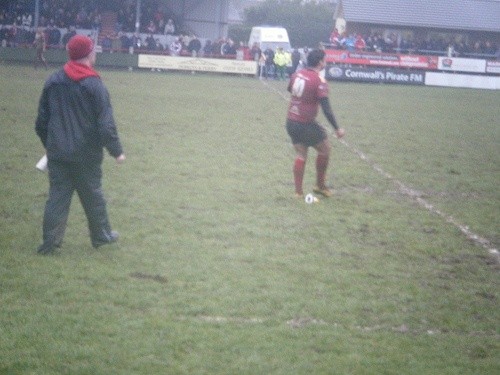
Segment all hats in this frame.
[67,34,95,61]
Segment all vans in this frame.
[248,26,293,76]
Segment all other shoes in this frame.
[91,231,119,249]
[313,186,333,197]
[39,245,60,255]
[293,193,306,199]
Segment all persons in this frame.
[273,46,281,81]
[188,34,201,56]
[151,38,162,72]
[169,36,182,55]
[286,49,293,78]
[250,41,262,78]
[292,50,300,72]
[285,51,344,203]
[203,39,213,57]
[303,46,309,54]
[136,36,148,54]
[320,30,500,56]
[34,34,127,251]
[242,40,251,76]
[0,0,242,59]
[263,44,275,81]
[277,47,287,81]
[144,32,155,54]
